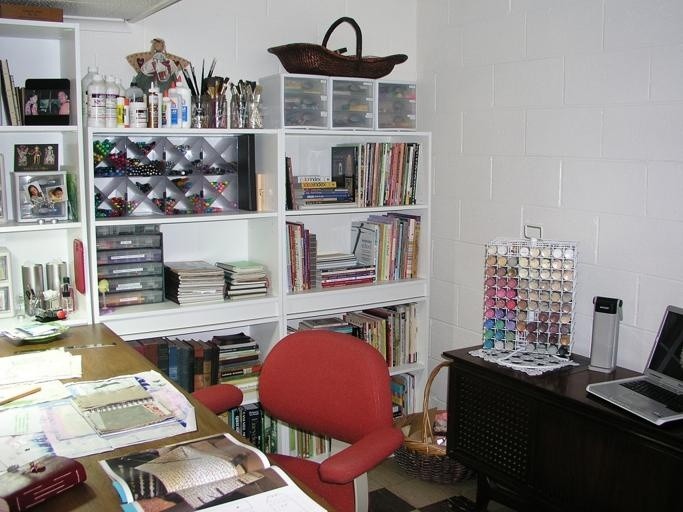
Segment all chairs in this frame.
[189,330,403,512]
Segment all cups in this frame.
[191,91,265,129]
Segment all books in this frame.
[287,212,421,294]
[288,303,418,367]
[65,375,177,438]
[0,452,87,511]
[94,430,330,512]
[9,74,20,126]
[285,143,421,212]
[164,260,269,306]
[289,425,330,459]
[14,85,21,124]
[0,58,24,126]
[122,332,289,465]
[391,373,414,419]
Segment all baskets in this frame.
[268,17,408,79]
[394,360,476,485]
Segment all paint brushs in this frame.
[180,58,263,129]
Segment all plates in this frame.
[19,321,71,343]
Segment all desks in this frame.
[0,323,332,512]
[438,341,683,510]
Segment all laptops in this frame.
[586,305,683,426]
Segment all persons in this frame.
[15,146,28,168]
[27,185,43,202]
[24,90,39,116]
[56,91,70,116]
[44,146,56,165]
[31,147,42,166]
[48,186,63,200]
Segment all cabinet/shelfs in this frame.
[1,18,433,466]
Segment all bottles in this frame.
[83,62,193,128]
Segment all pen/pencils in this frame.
[93,139,239,217]
[0,385,41,406]
